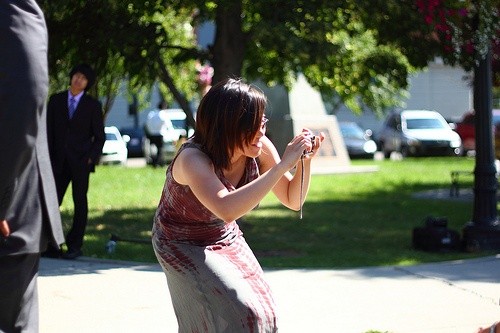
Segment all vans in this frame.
[142,108,197,166]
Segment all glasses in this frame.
[261,118,269,129]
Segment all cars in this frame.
[378,109,463,158]
[336,121,377,160]
[454,112,500,157]
[97,126,131,166]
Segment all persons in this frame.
[0,1,65,332]
[152,79,324,333]
[47,64,106,259]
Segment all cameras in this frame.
[301,132,316,156]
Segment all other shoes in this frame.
[62,249,83,260]
[41,248,63,257]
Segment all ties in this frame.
[69,97,76,120]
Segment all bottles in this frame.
[106,238,116,257]
